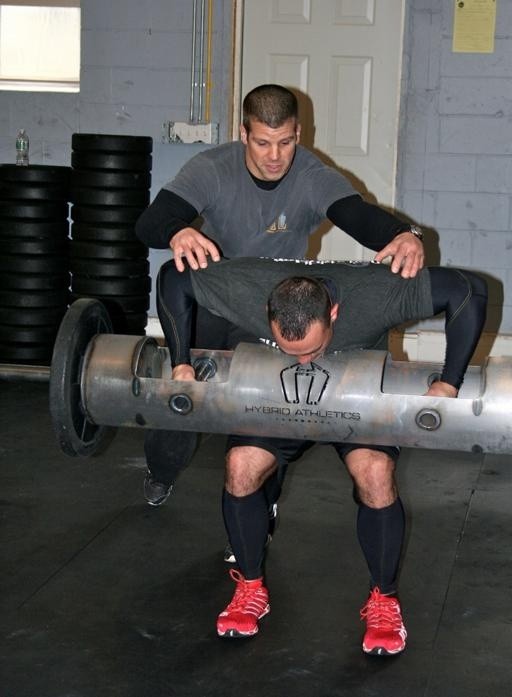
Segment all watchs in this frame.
[403,225,425,241]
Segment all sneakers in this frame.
[362,589,408,659]
[216,569,272,640]
[142,470,173,506]
[224,504,278,563]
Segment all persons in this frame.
[135,82,426,563]
[155,254,488,658]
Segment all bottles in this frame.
[15,128,30,165]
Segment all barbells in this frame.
[1,298,512,456]
